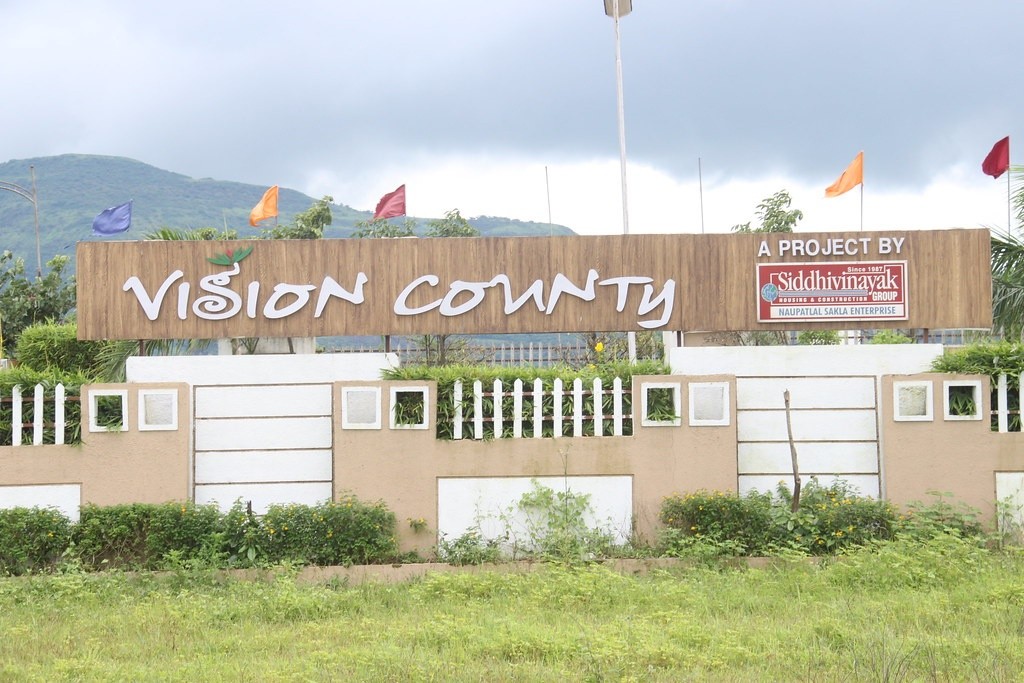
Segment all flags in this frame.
[825,151,863,196]
[373,184,406,220]
[92,201,132,236]
[249,185,279,226]
[981,135,1009,179]
